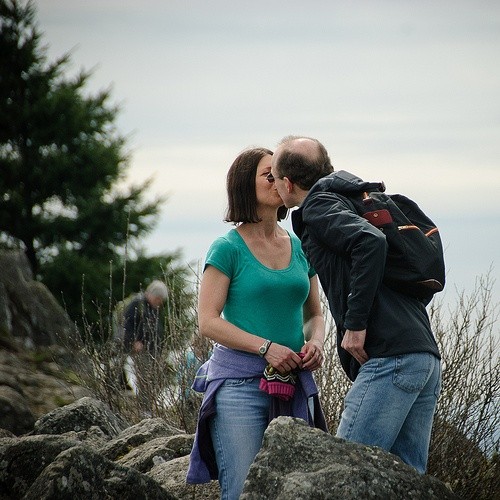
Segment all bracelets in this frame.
[263,341,273,357]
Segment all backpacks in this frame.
[305,190,446,294]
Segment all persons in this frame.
[266,137,444,473]
[198,149,328,500]
[122,280,223,404]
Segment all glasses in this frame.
[266,172,285,184]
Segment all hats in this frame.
[259,352,311,400]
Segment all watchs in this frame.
[258,340,271,357]
